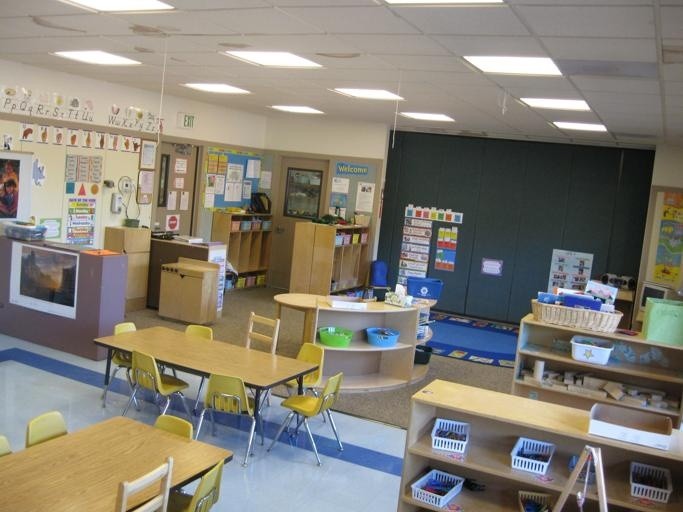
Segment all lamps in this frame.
[281,342,327,425]
[120,349,194,419]
[171,324,207,411]
[101,321,151,414]
[265,371,347,468]
[243,310,280,408]
[0,406,237,511]
[194,369,258,468]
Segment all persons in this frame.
[0,162,18,218]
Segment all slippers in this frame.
[251,193,271,213]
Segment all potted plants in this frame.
[281,167,323,221]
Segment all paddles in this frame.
[123,288,516,431]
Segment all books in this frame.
[407,276,442,299]
[638,296,682,348]
[418,307,430,324]
[417,324,429,339]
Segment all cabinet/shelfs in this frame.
[361,288,369,300]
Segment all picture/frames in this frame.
[91,323,322,434]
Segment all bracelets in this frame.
[124,218,139,227]
[366,327,400,346]
[319,326,352,346]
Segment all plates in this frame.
[209,209,268,289]
[309,221,370,297]
[396,379,682,512]
[0,236,129,361]
[288,220,313,298]
[507,312,682,429]
[103,226,151,312]
[274,292,434,396]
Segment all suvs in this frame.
[370,259,390,287]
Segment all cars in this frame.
[372,287,385,300]
[533,359,545,380]
